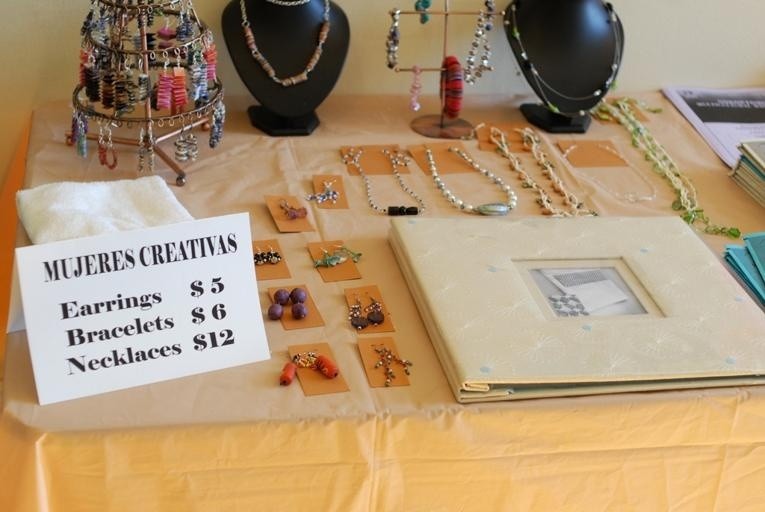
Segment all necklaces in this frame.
[598,97,741,238]
[240,0,330,87]
[504,1,621,117]
[341,147,426,215]
[562,142,657,203]
[425,144,517,214]
[488,127,597,217]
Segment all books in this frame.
[728,140,765,207]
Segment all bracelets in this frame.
[440,56,463,120]
[463,10,493,85]
[409,66,421,112]
[415,0,432,25]
[386,8,400,69]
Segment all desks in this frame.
[0,86,764,512]
